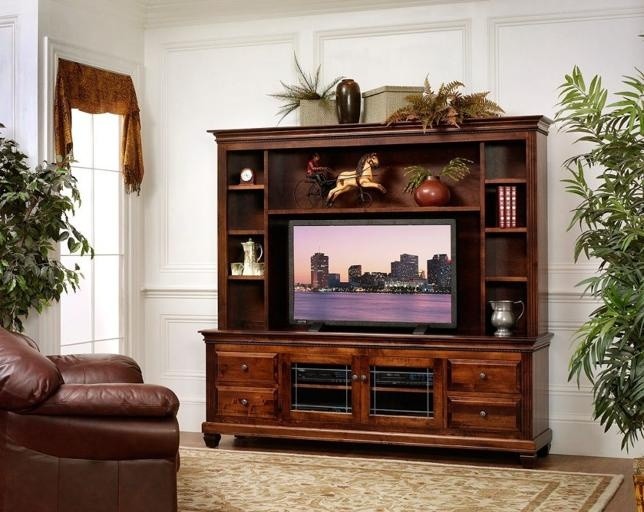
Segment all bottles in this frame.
[334,78,362,123]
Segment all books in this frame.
[498,185,517,228]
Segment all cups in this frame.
[253,263,265,276]
[230,261,243,275]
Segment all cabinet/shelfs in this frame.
[198,115,555,469]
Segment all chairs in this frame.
[0,326,180,512]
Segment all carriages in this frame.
[293,153,387,211]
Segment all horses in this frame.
[323,152,387,208]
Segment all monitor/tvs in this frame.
[289,219,456,334]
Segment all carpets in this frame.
[178,447,624,512]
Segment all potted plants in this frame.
[266,50,344,126]
[551,35,644,512]
[402,157,474,207]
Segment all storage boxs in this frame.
[362,86,424,123]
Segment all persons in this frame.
[306,153,329,187]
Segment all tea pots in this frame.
[487,299,523,337]
[240,237,262,275]
